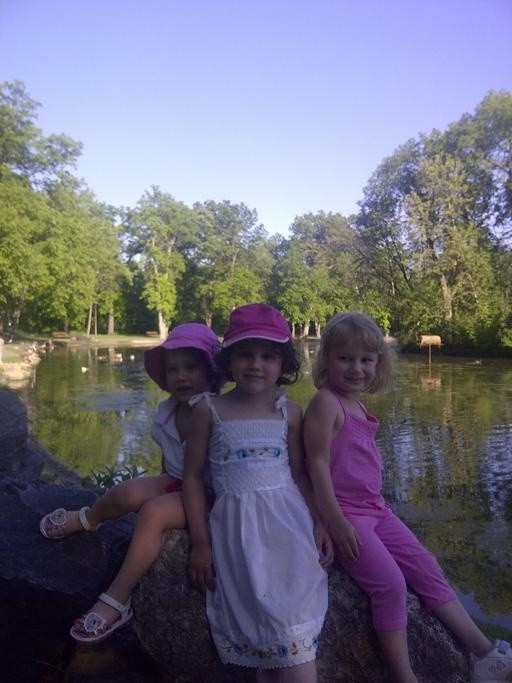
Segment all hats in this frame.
[222,304,291,348]
[144,323,222,395]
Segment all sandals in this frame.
[40,506,102,539]
[70,593,133,644]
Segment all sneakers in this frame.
[468,640,511,682]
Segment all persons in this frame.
[39,324,214,644]
[301,311,511,683]
[180,302,334,683]
